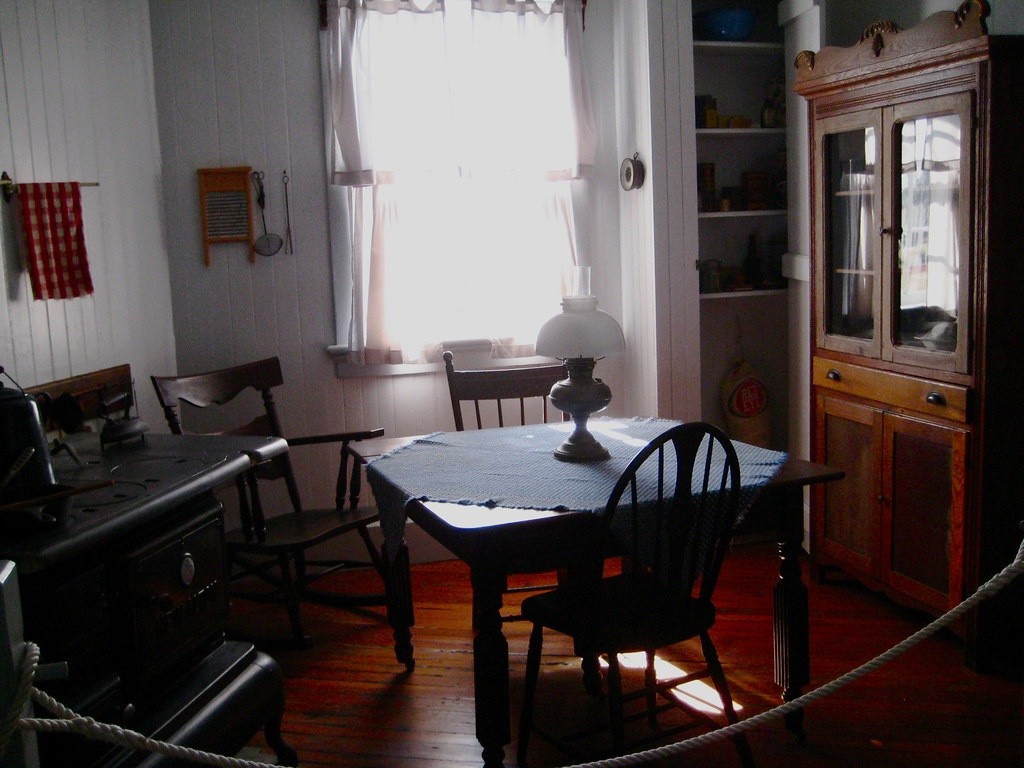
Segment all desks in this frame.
[0,433,290,641]
[345,415,846,768]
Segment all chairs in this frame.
[513,421,754,768]
[442,352,602,622]
[149,355,380,650]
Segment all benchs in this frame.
[38,648,299,768]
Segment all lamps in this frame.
[536,298,626,462]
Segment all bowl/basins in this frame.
[693,6,759,42]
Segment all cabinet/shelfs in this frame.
[694,39,787,299]
[792,0,1024,636]
